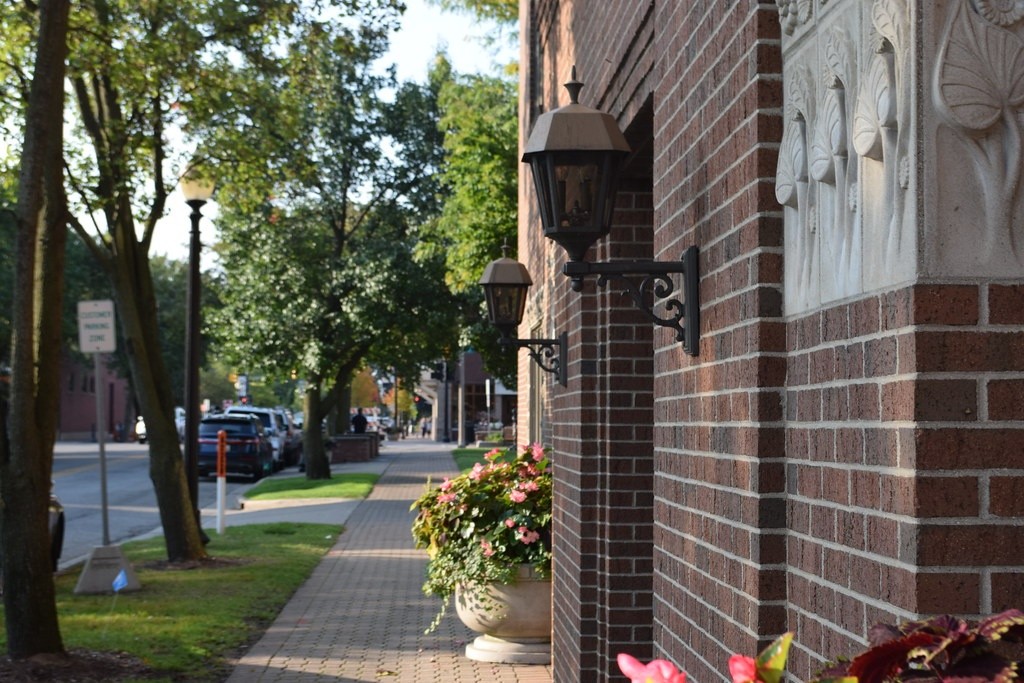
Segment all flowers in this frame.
[617,609,1024,683]
[410,443,553,636]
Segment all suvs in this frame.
[221,406,288,471]
[196,413,275,484]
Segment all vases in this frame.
[456,562,552,664]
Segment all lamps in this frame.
[478,237,567,388]
[521,64,701,357]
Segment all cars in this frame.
[136,407,185,444]
[270,408,304,464]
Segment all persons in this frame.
[351,407,368,433]
[419,416,428,438]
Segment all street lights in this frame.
[181,153,215,549]
